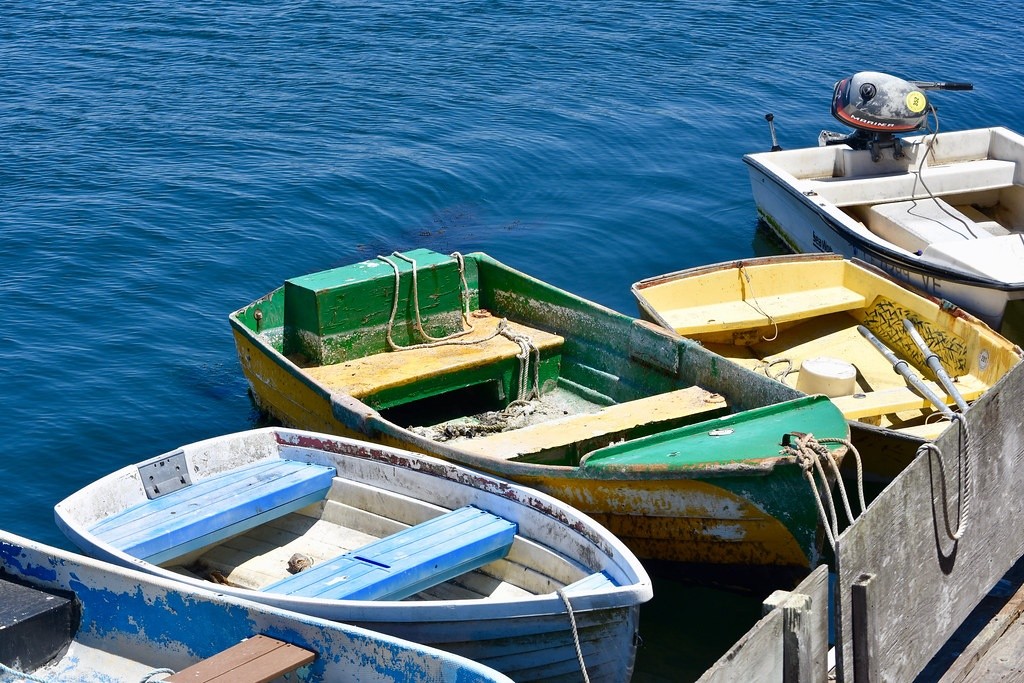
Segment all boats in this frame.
[741,70,1024,328]
[228,246,854,572]
[54,425,655,683]
[0,526,521,683]
[630,250,1024,445]
